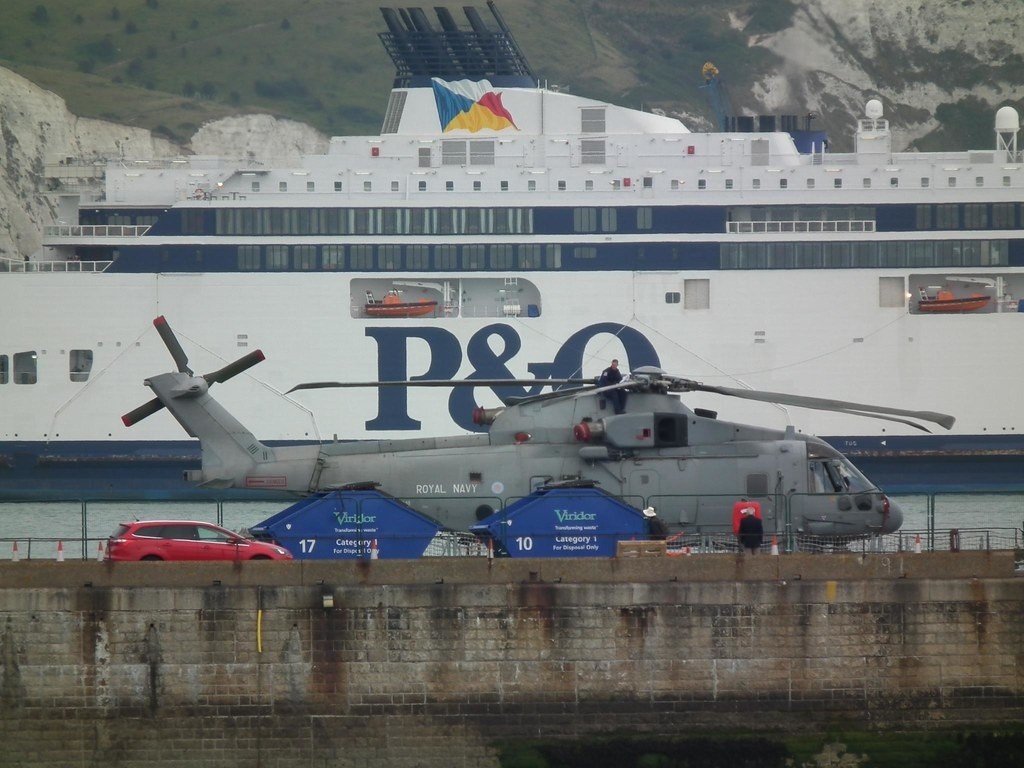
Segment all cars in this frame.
[103,519,294,561]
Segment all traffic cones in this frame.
[57,539,65,563]
[912,534,923,554]
[97,540,105,562]
[11,538,20,561]
[772,535,781,556]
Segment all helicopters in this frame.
[122,310,954,542]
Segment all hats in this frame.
[643,507,656,517]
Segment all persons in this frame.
[600,359,628,414]
[643,506,669,540]
[198,190,204,200]
[739,508,763,555]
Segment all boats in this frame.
[919,289,991,311]
[365,299,438,316]
[1,2,1023,485]
